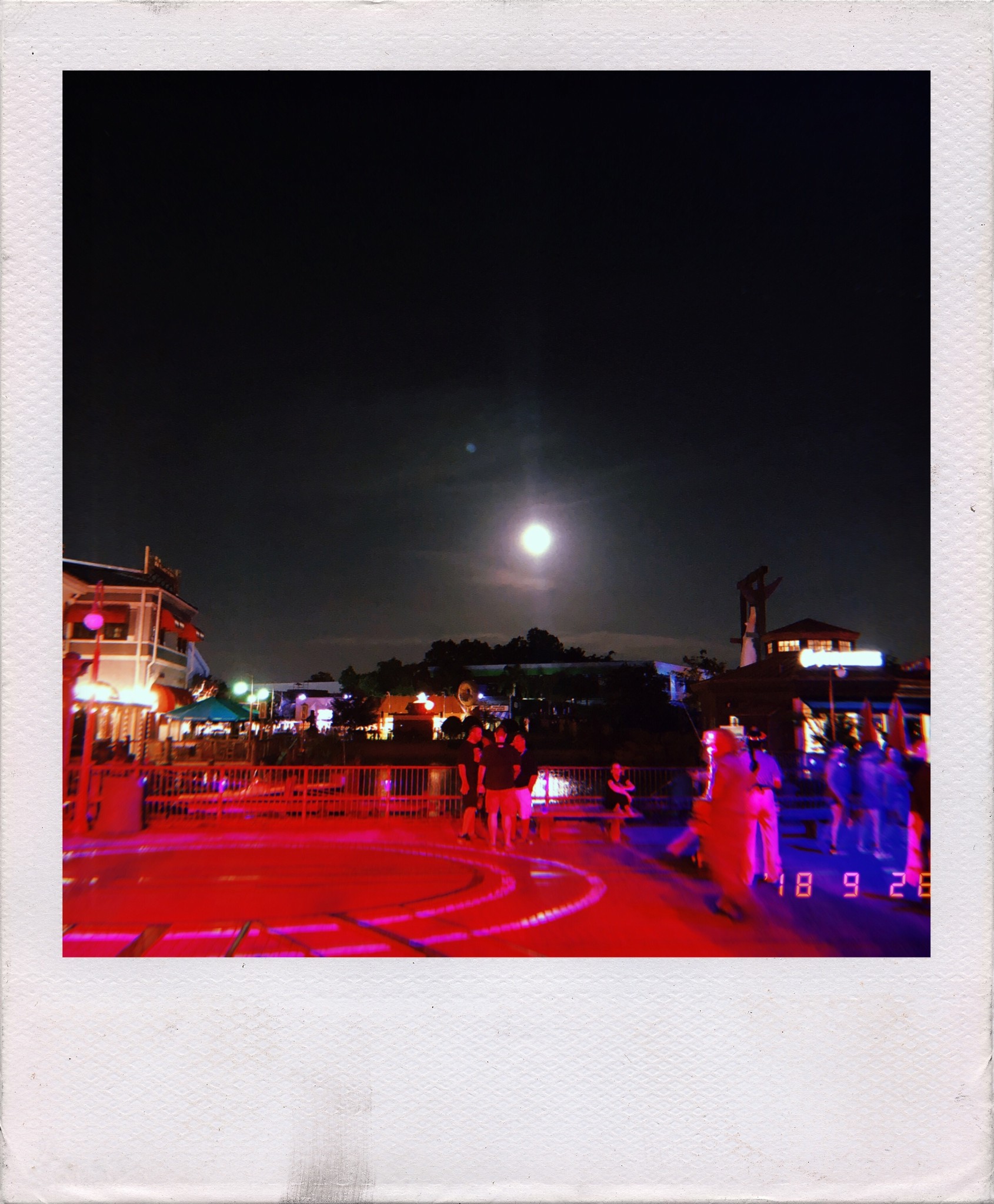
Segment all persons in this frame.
[665,724,784,920]
[457,722,539,853]
[603,763,636,813]
[823,741,933,903]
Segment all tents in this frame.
[164,695,266,739]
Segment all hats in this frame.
[744,726,766,741]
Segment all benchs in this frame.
[530,805,644,842]
[772,796,834,839]
